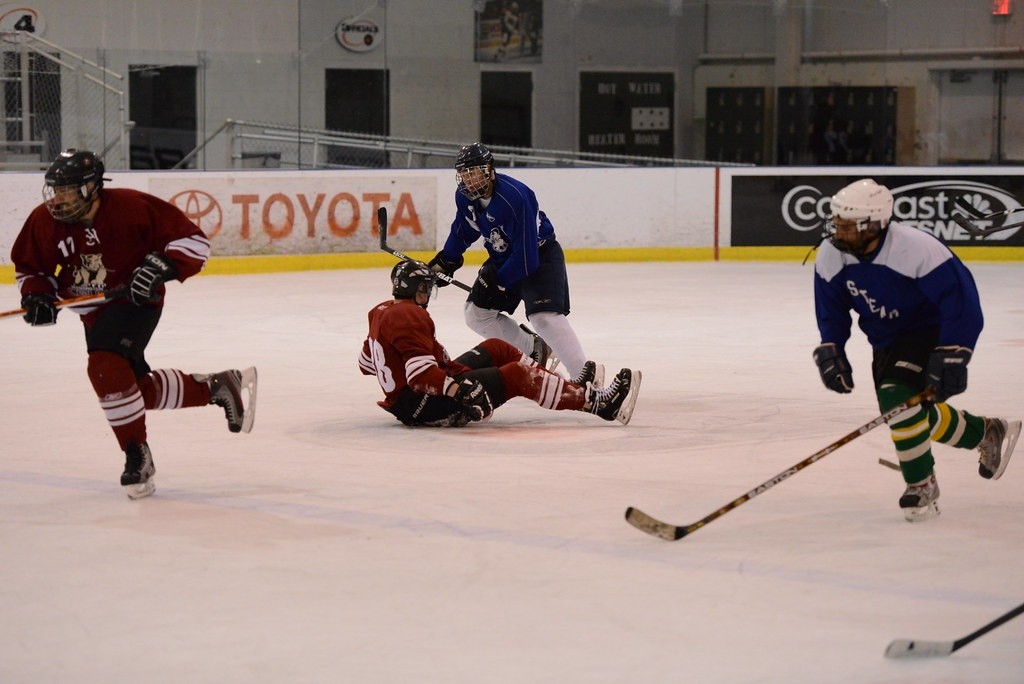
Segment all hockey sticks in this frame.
[884,602,1024,660]
[0,287,128,318]
[376,205,473,292]
[624,386,934,542]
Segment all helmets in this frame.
[454,143,496,181]
[829,178,894,232]
[391,260,435,298]
[44,148,112,186]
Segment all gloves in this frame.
[429,251,464,288]
[128,251,179,308]
[454,377,493,422]
[21,293,62,327]
[920,346,973,403]
[471,265,510,309]
[812,342,854,394]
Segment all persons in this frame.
[812,179,1022,523]
[357,260,642,427]
[11,147,258,500]
[423,141,585,383]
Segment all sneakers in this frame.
[584,368,643,425]
[568,361,605,391]
[899,473,943,522]
[518,323,560,372]
[977,417,1022,480]
[190,366,258,433]
[120,442,157,500]
